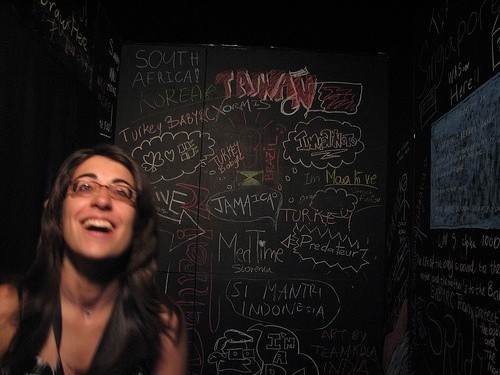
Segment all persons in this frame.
[0,142,191,375]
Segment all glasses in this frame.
[66,178,140,202]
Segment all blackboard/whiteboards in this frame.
[383,1,500,375]
[93,14,123,146]
[14,0,97,93]
[112,39,390,375]
